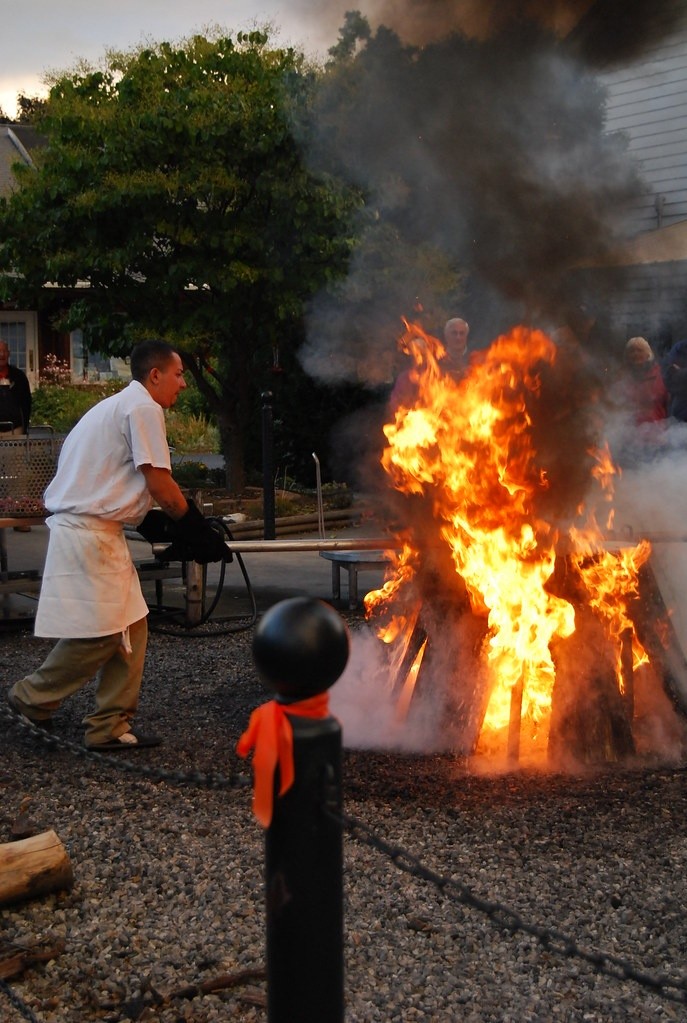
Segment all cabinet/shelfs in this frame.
[0,518,52,616]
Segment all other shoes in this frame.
[8,698,54,732]
[12,525,31,532]
[88,730,166,750]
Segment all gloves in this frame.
[138,510,176,561]
[171,500,233,564]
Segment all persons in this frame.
[5,341,233,754]
[662,337,687,422]
[443,317,471,368]
[616,336,667,454]
[0,341,34,534]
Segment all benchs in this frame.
[151,535,398,626]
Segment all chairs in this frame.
[135,507,256,636]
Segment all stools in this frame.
[321,548,391,616]
